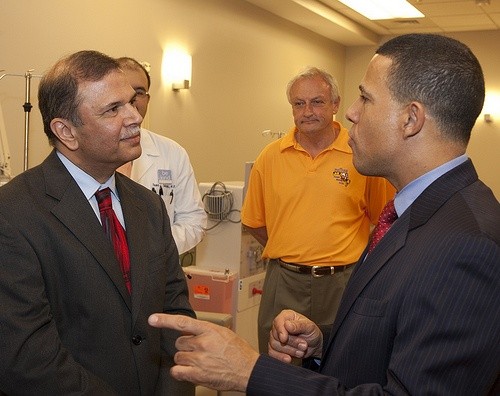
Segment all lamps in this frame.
[161,48,193,92]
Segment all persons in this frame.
[0,50,198,396]
[115,57,208,256]
[239,67,401,371]
[147,33,500,396]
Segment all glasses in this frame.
[136,90,149,97]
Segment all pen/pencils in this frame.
[169,190,174,204]
[159,186,163,197]
[152,188,157,194]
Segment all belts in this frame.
[279,259,354,277]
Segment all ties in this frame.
[94,188,132,297]
[368,199,398,255]
